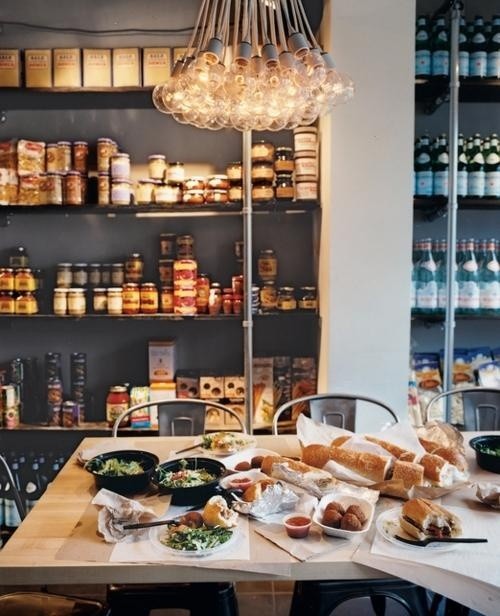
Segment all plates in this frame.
[376,507,468,553]
[194,432,258,455]
[218,469,302,504]
[148,511,238,557]
[221,449,283,473]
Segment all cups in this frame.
[283,513,313,538]
[227,474,254,490]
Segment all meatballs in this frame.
[325,503,345,517]
[347,505,366,526]
[251,456,266,468]
[236,462,250,471]
[341,514,362,531]
[183,512,204,528]
[322,510,341,528]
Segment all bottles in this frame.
[430,142,434,153]
[15,292,33,314]
[431,25,436,44]
[416,15,431,78]
[48,402,63,426]
[468,134,485,197]
[277,289,281,310]
[419,239,424,261]
[108,287,123,314]
[63,401,78,427]
[197,274,210,313]
[296,176,318,199]
[439,239,458,312]
[459,16,470,79]
[81,175,88,203]
[48,460,60,483]
[275,147,294,175]
[112,264,124,287]
[481,141,485,156]
[45,352,60,380]
[235,261,244,275]
[480,241,487,268]
[433,17,450,78]
[18,455,26,485]
[495,240,500,263]
[468,22,474,37]
[293,151,318,176]
[475,241,480,263]
[107,386,130,428]
[433,134,448,196]
[302,287,317,310]
[223,295,233,314]
[10,247,28,268]
[10,382,23,405]
[93,288,107,314]
[417,238,438,314]
[206,190,228,203]
[167,162,184,183]
[485,137,491,158]
[416,135,434,197]
[485,22,492,38]
[280,287,297,312]
[9,360,24,383]
[468,138,473,161]
[62,176,66,205]
[457,134,468,197]
[412,261,417,310]
[252,162,274,182]
[0,268,15,291]
[47,380,63,404]
[208,289,222,314]
[156,184,183,204]
[58,456,65,466]
[73,142,88,173]
[56,263,73,287]
[46,173,62,204]
[258,249,279,280]
[297,288,305,309]
[0,292,15,314]
[122,283,140,314]
[71,353,87,382]
[182,191,204,204]
[38,455,48,491]
[0,370,9,385]
[233,295,243,313]
[276,176,294,200]
[458,237,480,316]
[232,276,243,295]
[158,260,174,285]
[57,142,71,172]
[72,382,85,403]
[434,138,439,154]
[89,263,101,288]
[5,460,26,531]
[227,161,242,180]
[111,153,130,178]
[18,402,23,421]
[67,288,85,315]
[173,258,197,313]
[252,140,275,161]
[15,269,37,292]
[223,288,232,295]
[98,172,110,204]
[3,408,19,428]
[161,286,174,312]
[78,404,84,425]
[148,155,166,179]
[73,264,88,288]
[210,282,221,289]
[111,179,133,205]
[0,386,15,407]
[112,141,117,152]
[457,239,460,263]
[228,182,243,201]
[0,473,5,529]
[160,234,177,259]
[178,235,193,259]
[207,175,229,189]
[488,14,500,79]
[293,127,319,151]
[54,288,69,315]
[434,239,441,262]
[260,282,276,310]
[102,264,112,287]
[479,238,500,315]
[470,14,488,78]
[24,459,44,515]
[65,171,82,205]
[463,141,468,155]
[182,176,205,190]
[485,134,500,197]
[252,181,274,202]
[136,179,156,205]
[140,283,158,314]
[155,181,163,188]
[125,254,143,282]
[97,138,112,172]
[233,242,243,258]
[46,144,59,170]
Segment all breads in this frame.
[332,436,419,473]
[368,480,408,499]
[302,445,391,484]
[401,498,462,540]
[419,454,451,484]
[263,457,333,494]
[392,461,424,495]
[244,481,273,502]
[415,438,467,474]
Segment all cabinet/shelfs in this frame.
[0,1,331,534]
[412,1,499,432]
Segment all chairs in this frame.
[111,400,248,439]
[272,393,402,440]
[424,386,499,615]
[0,452,106,615]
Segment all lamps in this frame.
[154,0,355,135]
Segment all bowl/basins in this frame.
[469,435,500,474]
[150,457,227,506]
[312,493,375,538]
[84,449,160,496]
